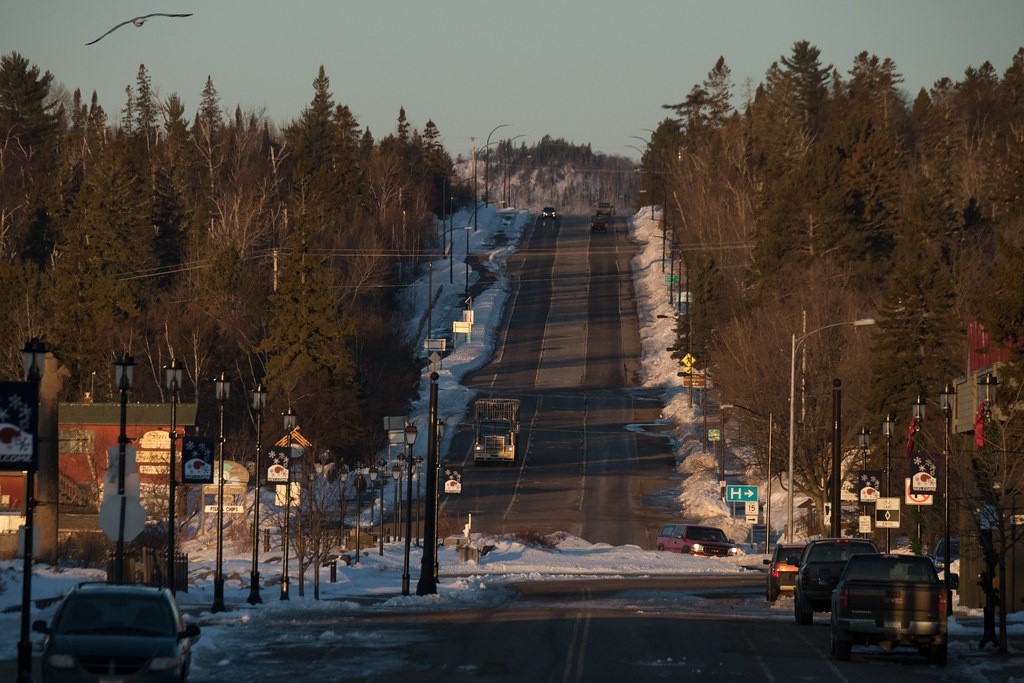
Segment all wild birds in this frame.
[84,13,194,46]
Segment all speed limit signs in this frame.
[745,502,759,515]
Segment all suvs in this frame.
[763,543,807,604]
[591,215,609,233]
[786,537,881,627]
[596,202,614,217]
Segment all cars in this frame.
[927,537,959,591]
[542,207,556,219]
[31,580,202,683]
[818,553,948,666]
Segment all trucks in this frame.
[473,397,522,467]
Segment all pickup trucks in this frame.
[656,523,736,559]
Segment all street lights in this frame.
[677,371,725,500]
[786,318,877,546]
[246,381,270,605]
[211,370,232,614]
[911,395,927,545]
[427,225,474,366]
[465,200,506,309]
[855,426,873,539]
[720,403,774,555]
[111,353,139,583]
[449,175,485,286]
[402,422,418,597]
[15,336,52,683]
[639,189,693,408]
[279,402,301,600]
[162,357,187,599]
[938,382,958,619]
[977,373,1001,649]
[474,120,532,232]
[882,413,897,554]
[340,418,446,585]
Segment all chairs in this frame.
[908,566,923,582]
[133,609,170,635]
